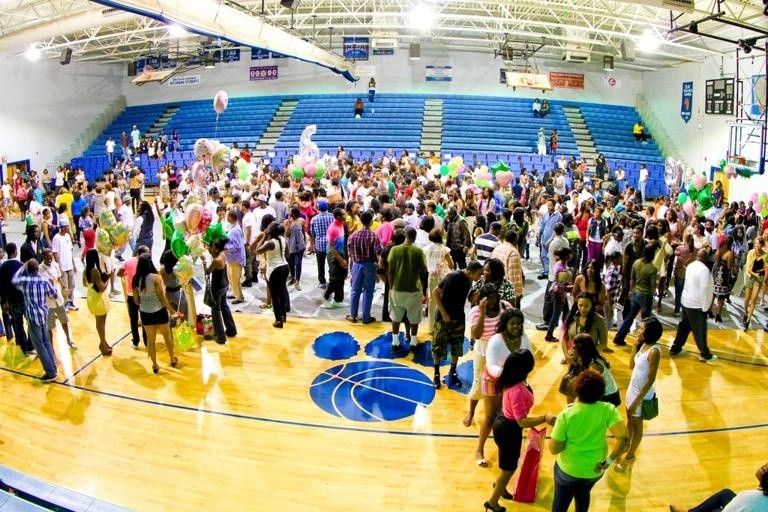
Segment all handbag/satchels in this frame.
[513,425,547,502]
[640,396,658,420]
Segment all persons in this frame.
[632,121,648,144]
[482,349,556,511]
[547,367,629,512]
[614,315,664,472]
[537,126,559,157]
[669,460,768,511]
[539,99,550,115]
[106,125,179,165]
[533,98,541,119]
[367,78,377,101]
[354,98,364,119]
[470,309,533,468]
[1,141,768,390]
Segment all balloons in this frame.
[213,91,229,114]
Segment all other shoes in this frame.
[99,315,237,375]
[63,301,79,314]
[228,277,345,327]
[109,288,125,299]
[23,338,77,382]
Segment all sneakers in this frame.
[346,314,356,322]
[698,353,718,363]
[450,371,462,388]
[433,372,441,389]
[670,347,686,355]
[613,338,627,346]
[391,334,424,354]
[363,316,376,324]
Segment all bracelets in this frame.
[606,456,613,463]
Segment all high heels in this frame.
[614,454,636,472]
[484,500,506,512]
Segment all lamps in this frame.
[408,44,421,59]
[738,38,751,54]
[763,0,768,15]
[203,53,215,68]
[688,20,698,33]
[503,46,514,62]
[621,40,635,62]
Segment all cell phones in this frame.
[600,459,606,466]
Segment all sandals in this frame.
[463,411,474,427]
[475,450,489,469]
[493,482,513,499]
